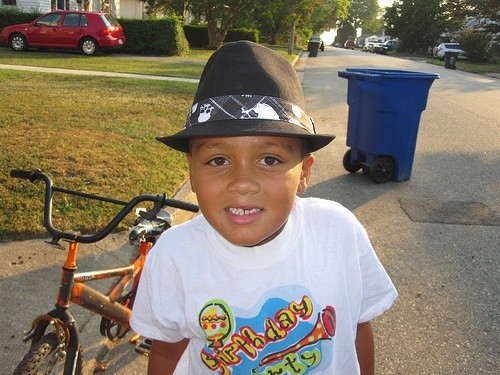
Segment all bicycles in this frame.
[8,169,199,375]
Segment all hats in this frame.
[155,39,336,154]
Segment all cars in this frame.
[437,42,469,60]
[365,40,398,54]
[344,40,354,50]
[0,10,126,56]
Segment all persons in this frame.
[131,40,398,375]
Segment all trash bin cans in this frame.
[307,41,320,57]
[444,51,461,70]
[337,66,441,184]
